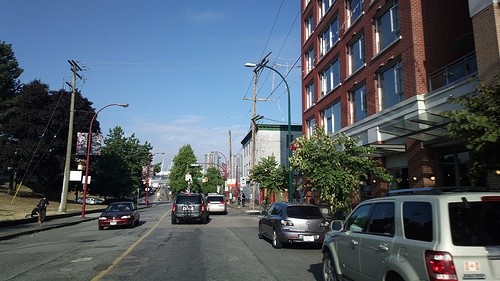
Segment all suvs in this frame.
[321,185,500,281]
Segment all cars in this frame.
[259,202,330,249]
[206,193,227,214]
[171,192,210,224]
[98,202,140,230]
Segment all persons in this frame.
[37,196,50,226]
[242,193,245,207]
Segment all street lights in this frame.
[82,103,129,217]
[211,151,227,177]
[244,63,293,204]
[145,153,164,208]
